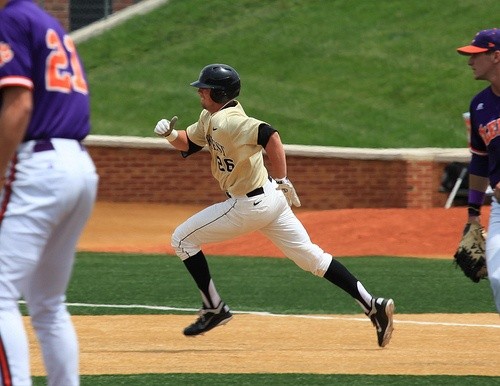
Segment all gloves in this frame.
[154,115,179,142]
[274,176,302,207]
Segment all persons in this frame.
[453,28,500,311]
[0,0,100,386]
[154,64,393,346]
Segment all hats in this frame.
[456,28,500,55]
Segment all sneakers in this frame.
[366,297,395,347]
[184,299,233,336]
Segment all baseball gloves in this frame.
[455,221,488,283]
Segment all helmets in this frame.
[190,64,241,100]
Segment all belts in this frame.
[34,140,84,152]
[226,175,273,198]
